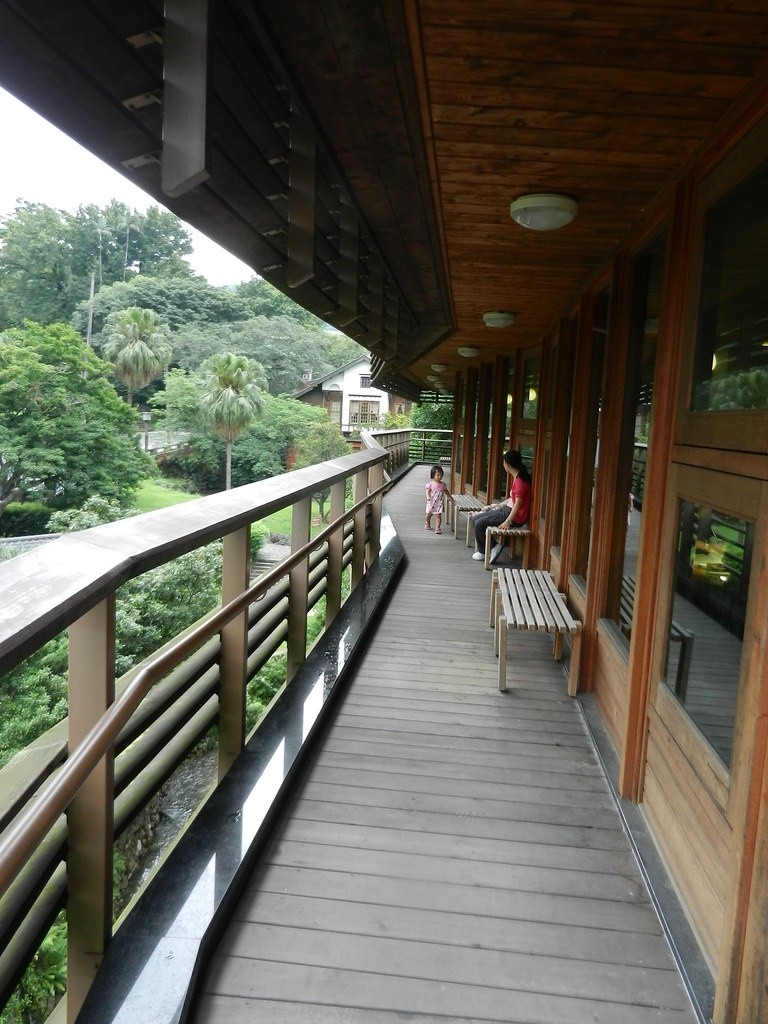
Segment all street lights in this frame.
[141,411,152,454]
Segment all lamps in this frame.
[431,364,447,372]
[427,375,448,394]
[482,312,514,328]
[458,347,479,358]
[511,194,578,230]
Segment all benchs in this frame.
[440,457,451,468]
[466,510,530,570]
[489,567,583,697]
[449,494,485,538]
[618,576,693,703]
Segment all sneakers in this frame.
[473,551,485,561]
[489,543,503,564]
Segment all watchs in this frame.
[506,519,512,524]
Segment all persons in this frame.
[626,493,634,544]
[471,450,531,564]
[425,466,455,534]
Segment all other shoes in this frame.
[425,523,431,529]
[435,528,442,534]
